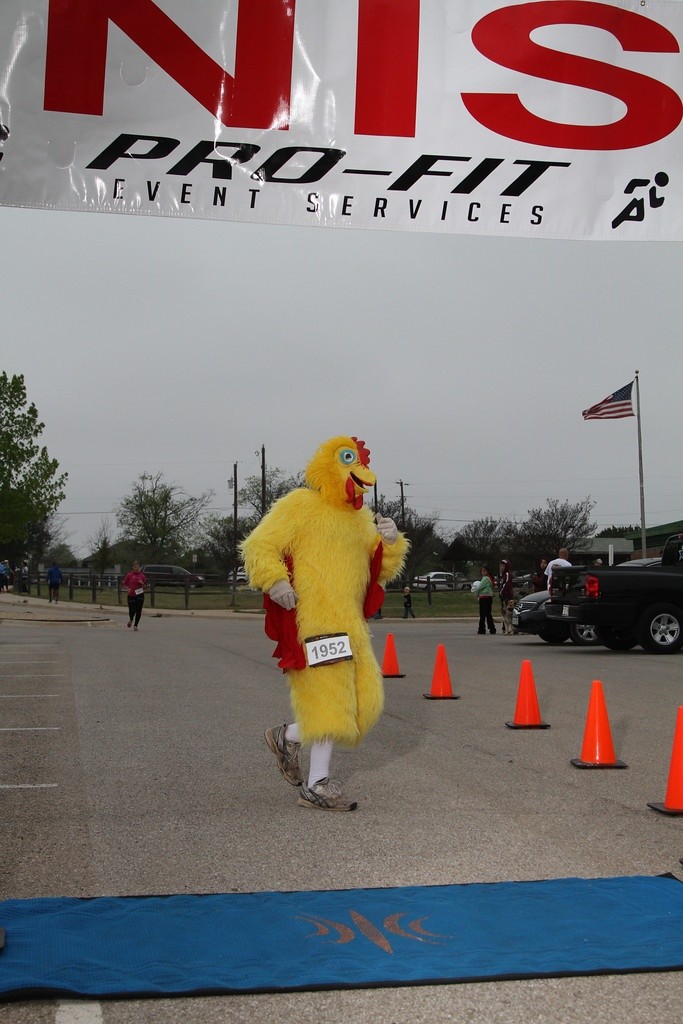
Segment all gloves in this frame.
[375,512,398,544]
[269,581,298,610]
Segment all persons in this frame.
[239,436,408,813]
[0,560,14,593]
[531,559,547,593]
[122,561,147,631]
[499,560,514,634]
[46,562,62,605]
[403,587,416,618]
[593,558,602,566]
[477,566,496,634]
[22,560,28,591]
[544,548,572,591]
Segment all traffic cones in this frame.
[505,660,551,728]
[569,680,629,767]
[423,644,461,699]
[647,706,683,813]
[380,633,406,678]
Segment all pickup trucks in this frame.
[545,533,683,654]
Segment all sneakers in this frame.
[298,777,358,811]
[264,722,304,786]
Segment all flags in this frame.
[581,380,635,420]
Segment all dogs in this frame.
[502,600,517,636]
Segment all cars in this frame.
[511,558,662,646]
[413,572,471,593]
[228,566,247,585]
[139,566,204,589]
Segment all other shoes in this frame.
[128,620,133,627]
[134,625,138,631]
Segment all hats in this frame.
[592,559,602,565]
